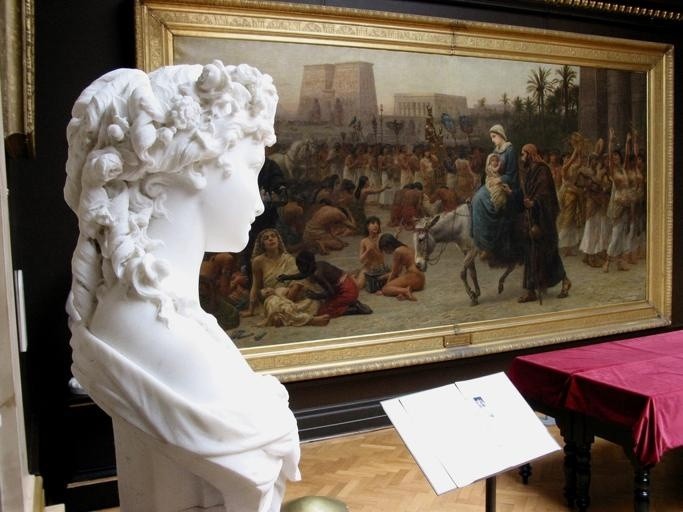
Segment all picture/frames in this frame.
[134,1,673,385]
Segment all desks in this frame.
[514,329,683,512]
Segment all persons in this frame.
[67,56,303,512]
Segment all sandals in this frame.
[559,279,570,299]
[518,293,536,302]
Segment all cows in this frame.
[257,158,291,207]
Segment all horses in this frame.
[410,203,517,307]
[264,138,310,180]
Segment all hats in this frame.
[490,124,506,140]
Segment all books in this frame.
[378,369,561,498]
